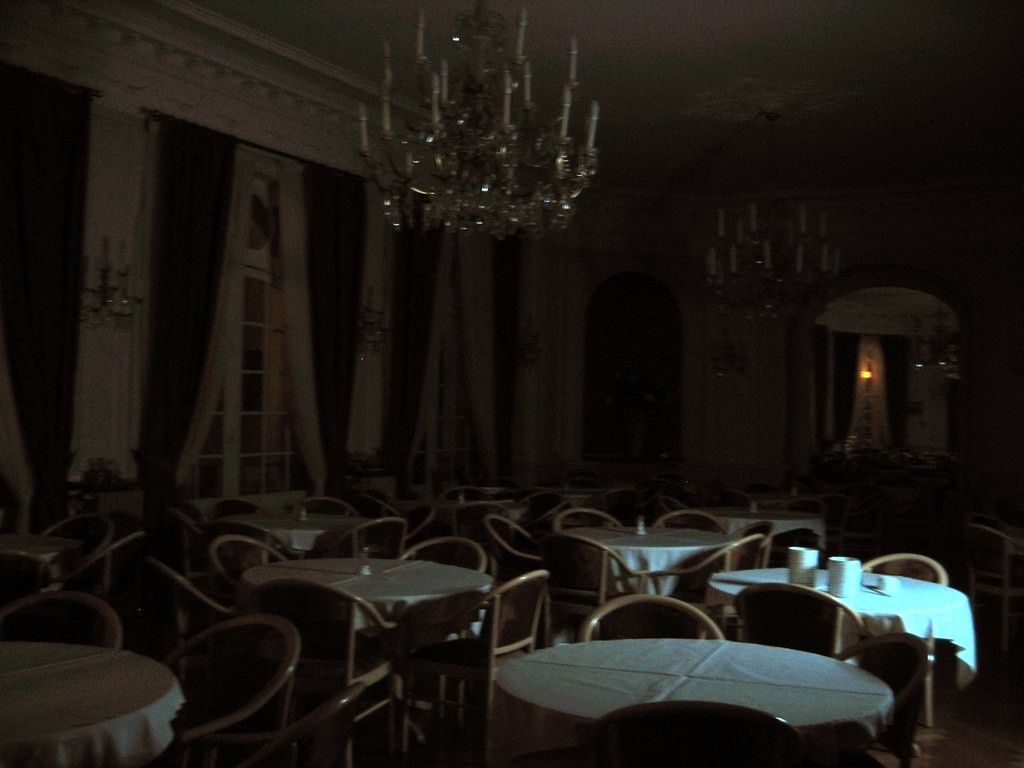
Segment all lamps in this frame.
[703,105,843,322]
[80,239,143,326]
[356,287,389,352]
[357,0,600,240]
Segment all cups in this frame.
[788,546,819,588]
[827,556,862,598]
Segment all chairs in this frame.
[0,470,1024,768]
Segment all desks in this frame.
[239,556,495,746]
[567,525,743,612]
[489,636,894,768]
[695,506,826,553]
[0,534,81,559]
[0,641,185,768]
[699,568,979,728]
[219,511,371,552]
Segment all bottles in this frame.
[633,515,648,535]
[749,500,760,514]
[298,506,308,522]
[358,546,372,576]
[456,492,466,505]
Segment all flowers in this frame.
[83,455,123,490]
